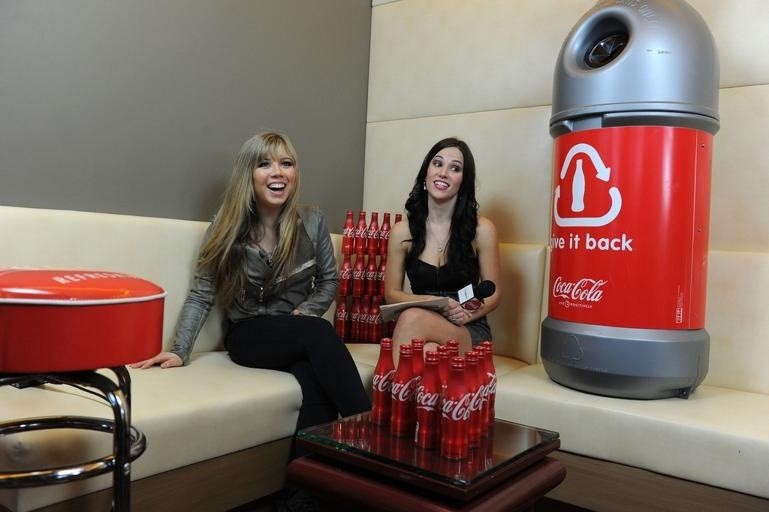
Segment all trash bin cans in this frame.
[541,0,720,400]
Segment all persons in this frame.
[384,138,503,368]
[125,131,371,499]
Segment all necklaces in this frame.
[425,215,451,287]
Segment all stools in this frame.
[1,269,167,511]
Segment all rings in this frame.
[452,313,456,320]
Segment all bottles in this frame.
[335,210,402,344]
[334,411,493,481]
[370,338,497,460]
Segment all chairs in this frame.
[323,229,545,377]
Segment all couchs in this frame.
[493,233,769,512]
[1,205,375,511]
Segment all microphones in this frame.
[456,280,496,311]
